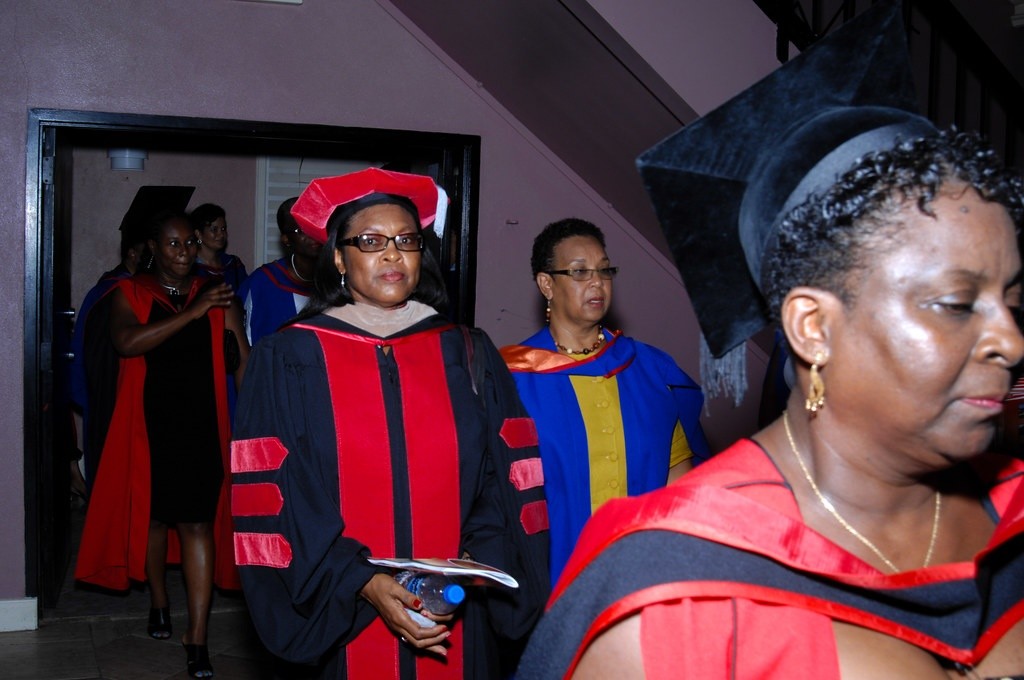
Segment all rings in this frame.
[401,636,409,643]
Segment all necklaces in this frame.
[161,278,187,296]
[291,253,312,282]
[783,408,939,572]
[555,325,605,355]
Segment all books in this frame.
[368,557,520,589]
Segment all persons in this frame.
[75,197,324,680]
[517,0,1024,680]
[497,220,717,589]
[232,168,552,680]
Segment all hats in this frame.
[289,167,450,248]
[118,185,196,240]
[635,0,1024,362]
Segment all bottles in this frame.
[392,570,465,615]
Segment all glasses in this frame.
[342,232,424,254]
[543,268,618,281]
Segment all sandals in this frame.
[182,643,214,680]
[148,604,172,639]
